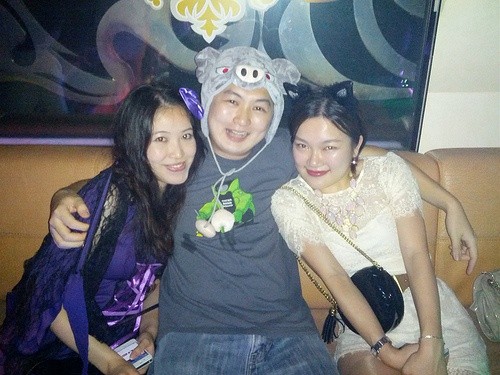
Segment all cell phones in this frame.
[113,337,152,371]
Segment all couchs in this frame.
[0,143,500,374]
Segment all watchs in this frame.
[370,337,392,356]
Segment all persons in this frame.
[1,81,205,375]
[268,80,491,375]
[48,47,477,375]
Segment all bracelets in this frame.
[419,334,444,342]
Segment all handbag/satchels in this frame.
[336,265,404,337]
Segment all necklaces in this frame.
[311,170,366,239]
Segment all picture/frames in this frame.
[0,0,445,153]
